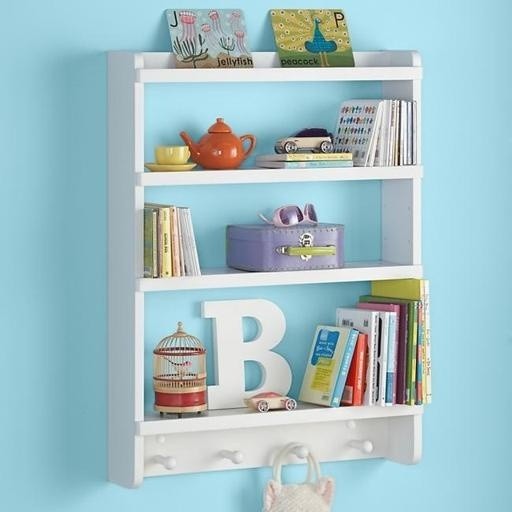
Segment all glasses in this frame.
[259,204,318,228]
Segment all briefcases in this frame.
[226,223,345,271]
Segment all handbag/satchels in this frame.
[262,442,335,512]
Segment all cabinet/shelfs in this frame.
[108,48,421,490]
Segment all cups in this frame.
[155,146,190,165]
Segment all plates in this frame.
[145,162,197,172]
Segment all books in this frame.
[255,159,354,169]
[331,100,418,167]
[143,202,201,279]
[298,278,432,408]
[255,153,353,162]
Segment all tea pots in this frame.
[179,117,256,169]
